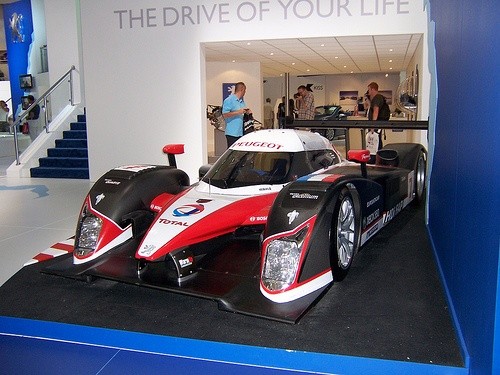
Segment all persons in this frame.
[25,95,40,141]
[0,101,9,131]
[363,81,384,164]
[222,82,250,149]
[263,84,316,132]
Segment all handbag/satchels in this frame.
[366,131,379,155]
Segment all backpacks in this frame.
[383,98,390,121]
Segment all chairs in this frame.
[264,158,288,184]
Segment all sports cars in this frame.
[310,104,349,141]
[38,119,430,325]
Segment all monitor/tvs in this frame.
[20,74,32,88]
